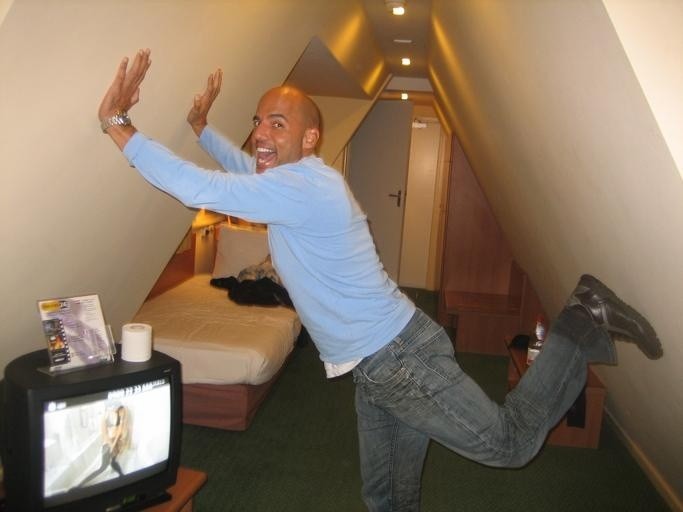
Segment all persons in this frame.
[69,406,129,488]
[96,49,662,512]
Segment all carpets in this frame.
[100,283,682,512]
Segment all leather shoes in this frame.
[567,274,663,361]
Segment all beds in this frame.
[124,273,304,433]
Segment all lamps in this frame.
[400,89,409,102]
[400,55,412,69]
[391,0,405,17]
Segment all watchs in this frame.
[100,111,132,134]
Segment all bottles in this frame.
[526,314,546,368]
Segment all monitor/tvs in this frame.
[1,343,183,512]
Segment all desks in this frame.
[503,328,605,452]
